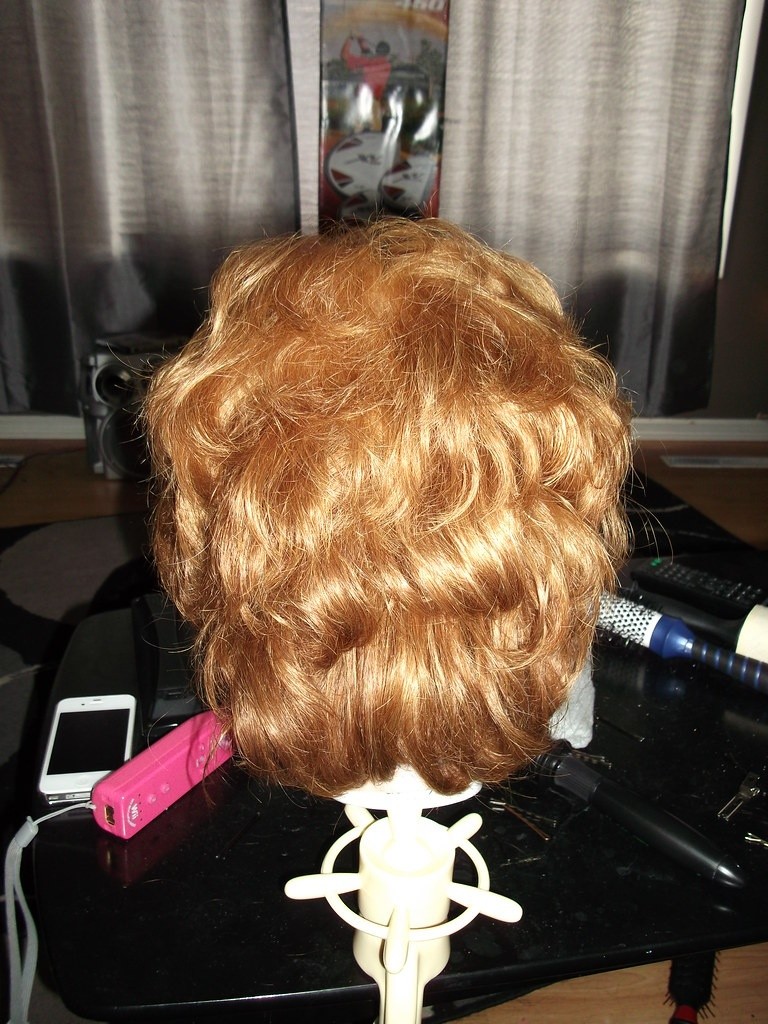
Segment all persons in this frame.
[340,28,395,135]
[138,216,631,801]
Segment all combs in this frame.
[584,589,768,697]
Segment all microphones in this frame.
[529,740,746,895]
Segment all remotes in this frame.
[629,557,768,618]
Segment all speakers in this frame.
[83,334,184,480]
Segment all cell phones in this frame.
[39,693,137,804]
